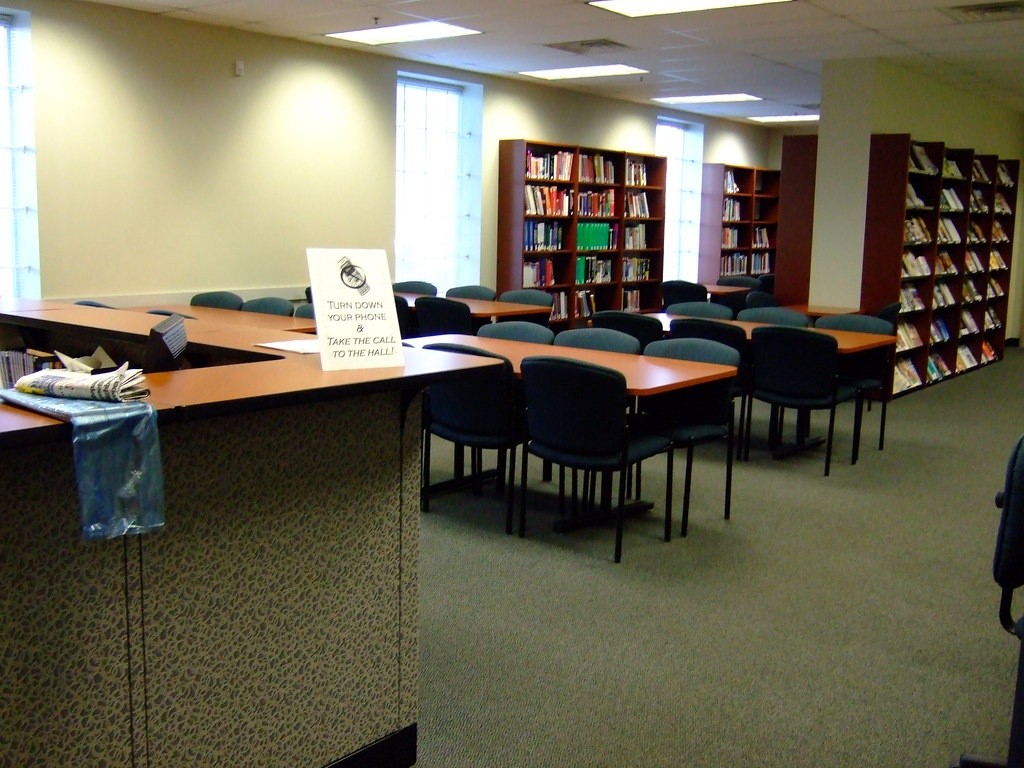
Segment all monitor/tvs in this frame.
[142,314,188,374]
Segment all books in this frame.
[522,150,650,321]
[719,170,770,276]
[893,146,1013,395]
[0,350,67,388]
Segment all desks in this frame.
[395,291,552,323]
[118,304,317,334]
[703,284,752,297]
[402,334,738,534]
[588,314,898,461]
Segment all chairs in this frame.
[75,281,894,564]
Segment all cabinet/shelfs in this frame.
[859,133,1021,399]
[698,133,818,305]
[498,139,668,328]
